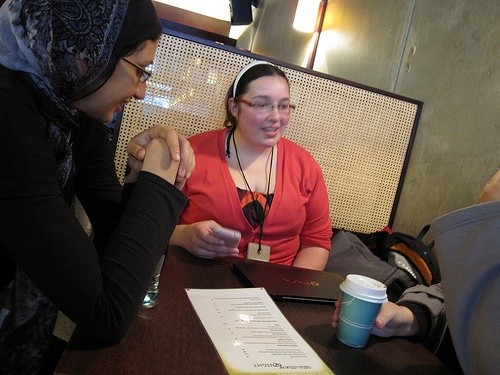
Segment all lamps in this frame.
[293,0,322,34]
[229,0,259,25]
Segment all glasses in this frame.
[236,98,296,115]
[119,56,152,82]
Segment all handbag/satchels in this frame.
[324,229,418,303]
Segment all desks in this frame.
[54,243,457,375]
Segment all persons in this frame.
[0,0,197,374]
[168,61,333,272]
[330,168,500,375]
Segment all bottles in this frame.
[142,246,167,309]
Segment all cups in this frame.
[335,274,388,348]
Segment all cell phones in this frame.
[213,225,241,248]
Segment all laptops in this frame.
[230,259,346,303]
